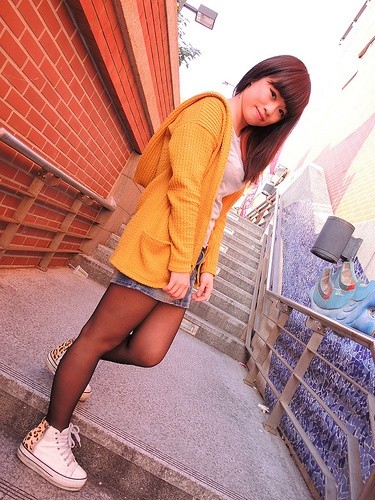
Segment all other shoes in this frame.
[17,417,88,492]
[46,338,93,401]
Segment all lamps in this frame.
[310,216,362,265]
[178,1,218,30]
[261,183,277,197]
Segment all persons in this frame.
[15,55,311,492]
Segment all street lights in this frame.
[183,2,219,30]
